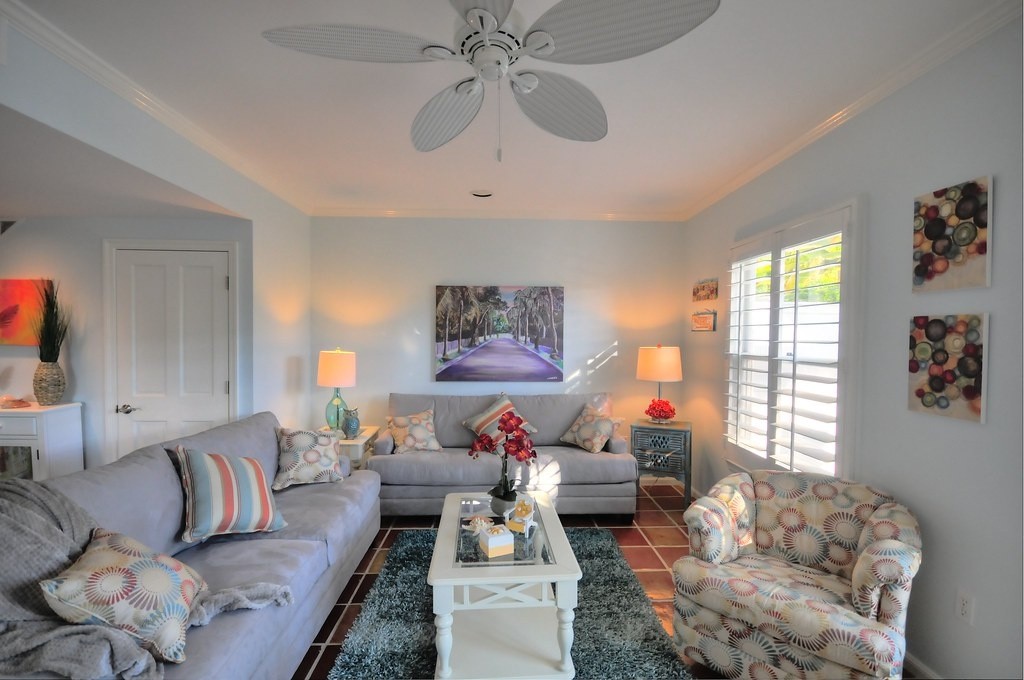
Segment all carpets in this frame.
[327,528,697,680]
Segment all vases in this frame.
[487,486,517,516]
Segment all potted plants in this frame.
[25,273,74,406]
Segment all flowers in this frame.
[644,399,677,420]
[467,411,537,493]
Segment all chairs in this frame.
[503,494,534,538]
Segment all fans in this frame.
[258,0,719,163]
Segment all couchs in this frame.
[671,469,923,680]
[366,392,639,526]
[0,411,382,680]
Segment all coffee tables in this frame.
[427,492,582,680]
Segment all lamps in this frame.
[316,347,357,432]
[635,344,682,423]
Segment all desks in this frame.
[0,401,83,482]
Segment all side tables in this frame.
[317,425,381,470]
[630,417,693,513]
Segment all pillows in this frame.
[37,527,210,664]
[559,402,626,454]
[385,409,443,455]
[272,426,344,491]
[176,444,289,543]
[461,391,538,455]
